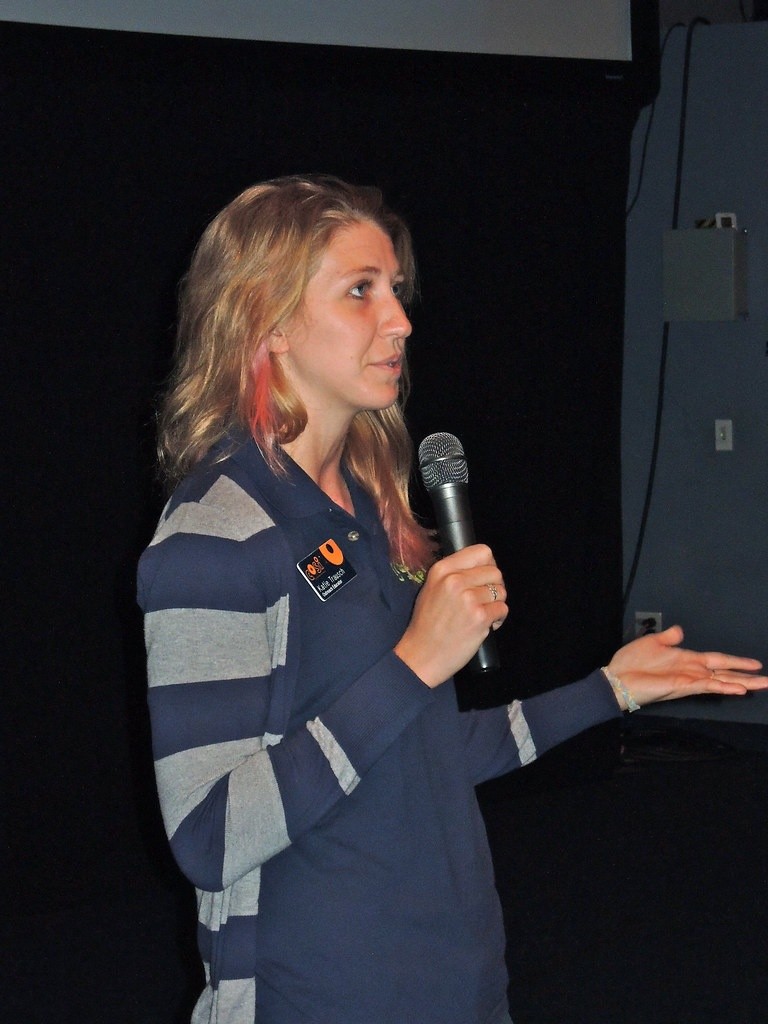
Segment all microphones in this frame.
[419,432,498,672]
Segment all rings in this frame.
[486,583,499,601]
[711,669,715,680]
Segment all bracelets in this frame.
[600,666,640,712]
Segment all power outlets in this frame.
[633,610,661,637]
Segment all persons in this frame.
[136,181,767,1024]
